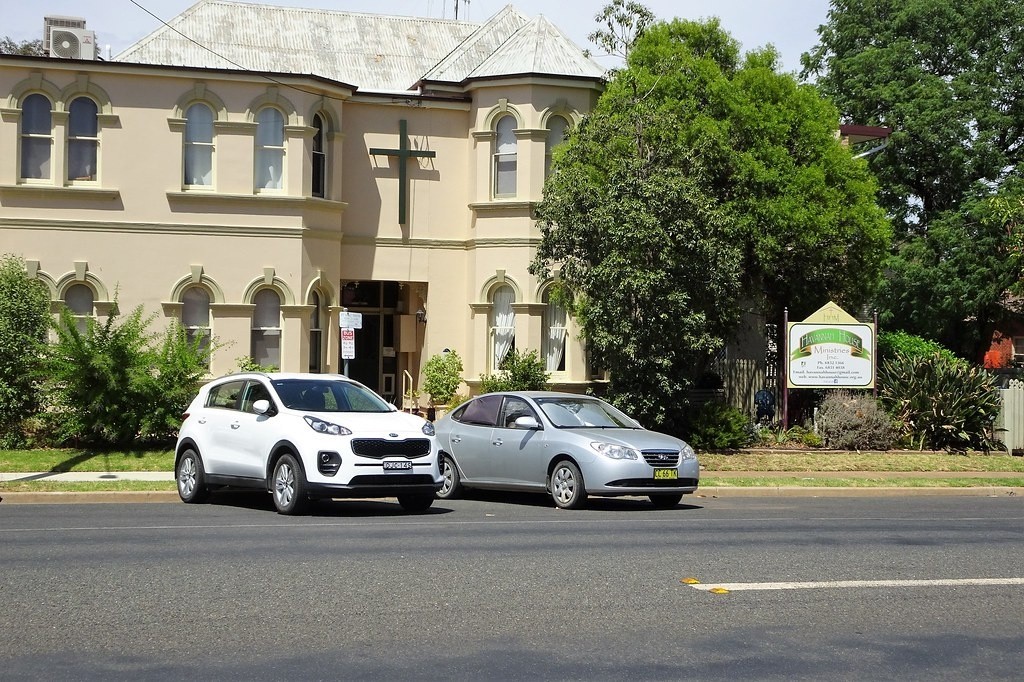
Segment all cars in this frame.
[432,389,700,510]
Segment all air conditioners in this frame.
[50,27,96,60]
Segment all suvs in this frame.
[172,371,446,514]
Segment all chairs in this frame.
[302,390,326,411]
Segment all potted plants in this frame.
[423,350,464,422]
[404,389,420,409]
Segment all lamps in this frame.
[417,309,426,327]
